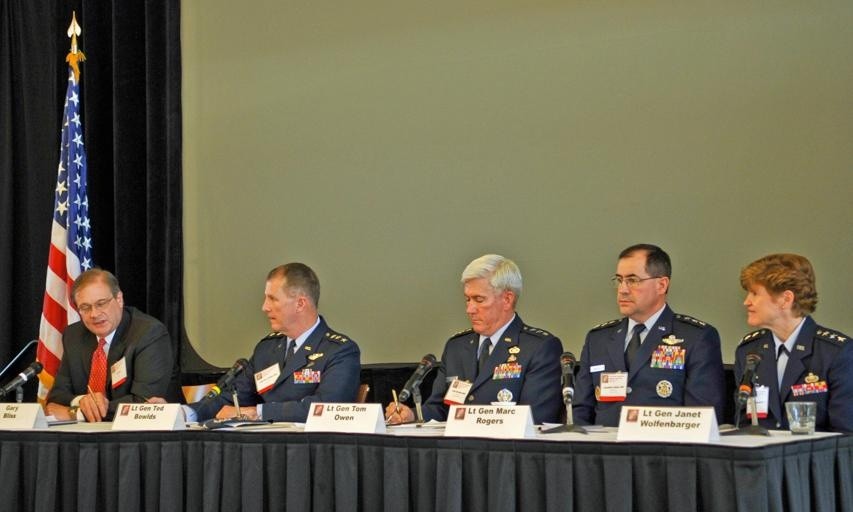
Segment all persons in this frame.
[570,241,728,427]
[731,251,853,435]
[43,268,174,424]
[143,261,362,425]
[383,252,565,425]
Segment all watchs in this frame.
[66,406,78,420]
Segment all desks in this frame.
[1,419,849,510]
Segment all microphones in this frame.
[559,351,576,404]
[201,358,250,404]
[1,362,43,397]
[737,351,763,406]
[399,354,436,401]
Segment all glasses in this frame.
[610,275,661,288]
[78,296,115,314]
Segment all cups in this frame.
[785,401,818,435]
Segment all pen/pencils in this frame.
[391,389,400,413]
[87,385,97,403]
[232,390,241,417]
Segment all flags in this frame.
[34,52,98,417]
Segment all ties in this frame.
[283,340,296,365]
[478,338,492,375]
[86,338,107,398]
[624,323,645,369]
